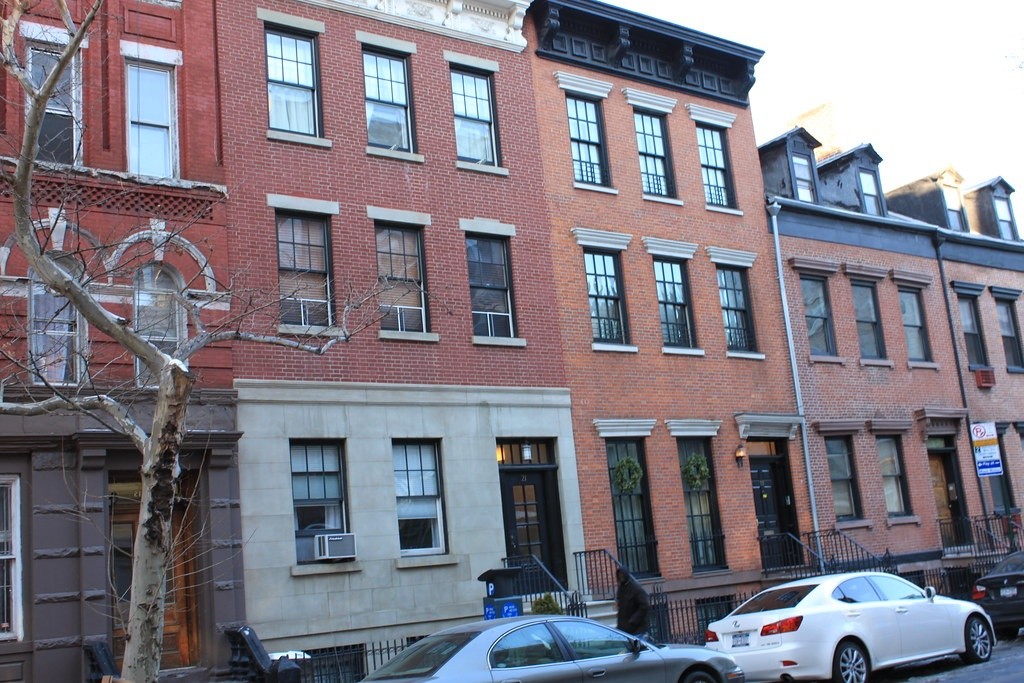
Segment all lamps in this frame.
[522,442,531,460]
[735,447,747,467]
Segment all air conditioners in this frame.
[315,532,358,559]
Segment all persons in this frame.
[614,568,650,644]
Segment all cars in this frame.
[358,615,745,683]
[703,571,998,683]
[971,550,1024,642]
